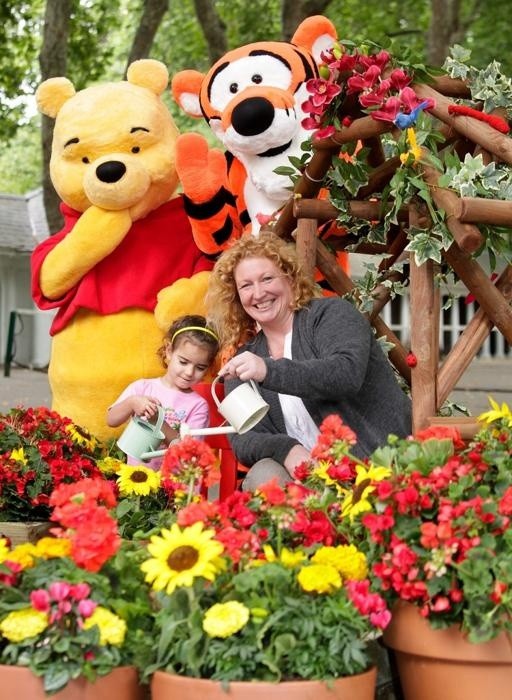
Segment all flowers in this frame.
[0,398,512,700]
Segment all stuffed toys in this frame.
[29,57,231,464]
[168,13,383,372]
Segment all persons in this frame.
[215,233,415,497]
[106,314,241,481]
